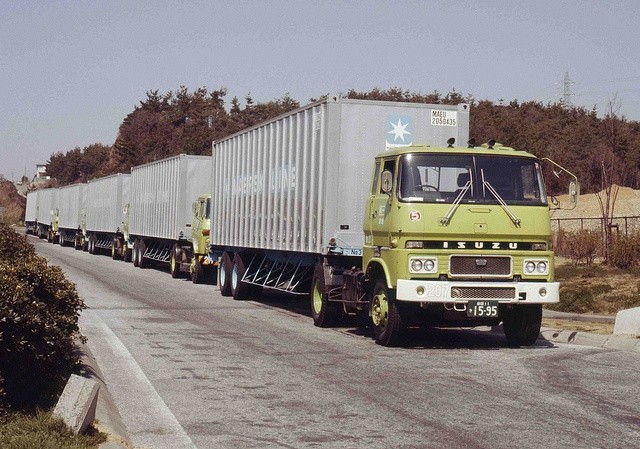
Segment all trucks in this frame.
[84,172,133,261]
[57,182,87,251]
[25,191,40,236]
[208,95,581,347]
[127,154,218,283]
[36,188,59,244]
